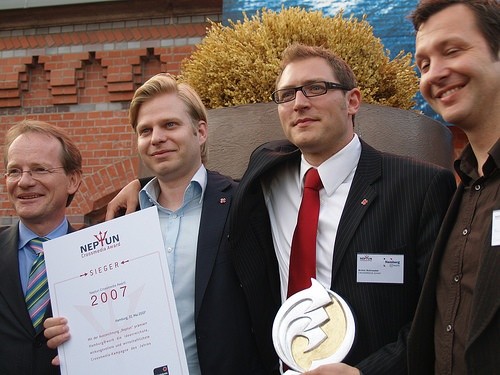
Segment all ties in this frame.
[23,239,50,334]
[283,168,322,373]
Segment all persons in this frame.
[105,48,458,375]
[0,114,85,375]
[44,72,245,375]
[399,0,500,375]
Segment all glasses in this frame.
[5,165,73,182]
[271,80,351,105]
